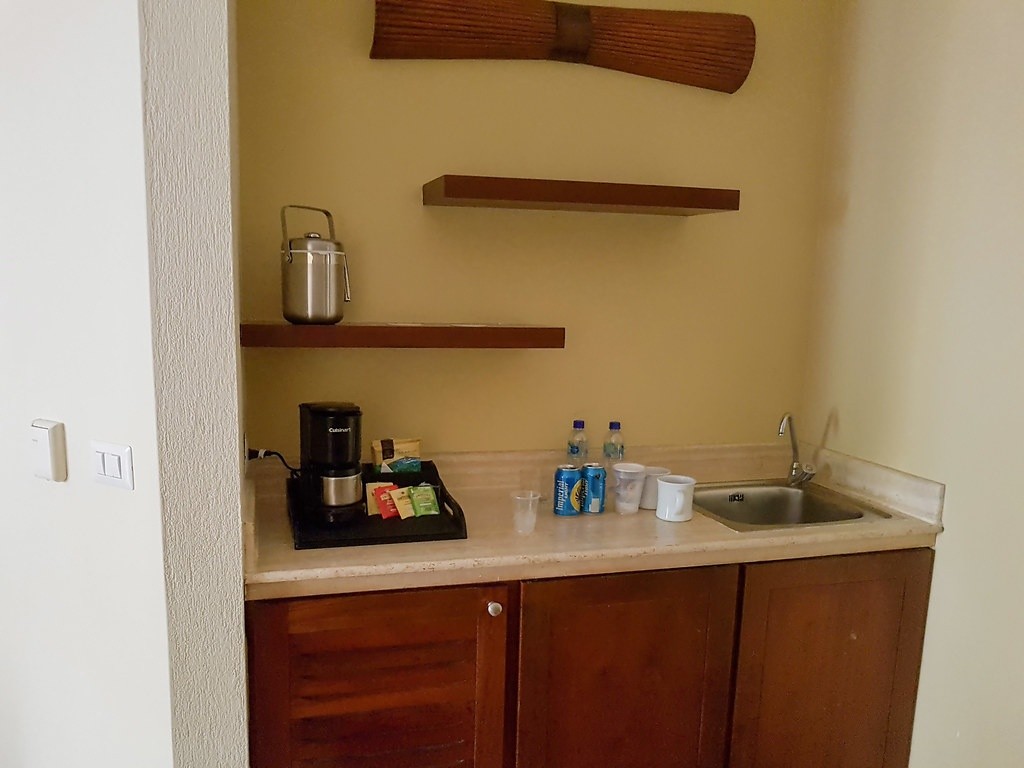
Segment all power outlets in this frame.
[244,431,248,475]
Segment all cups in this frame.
[611,463,648,513]
[639,467,671,509]
[509,490,541,534]
[656,475,696,522]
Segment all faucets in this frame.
[778,412,819,484]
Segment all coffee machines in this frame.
[299,402,365,526]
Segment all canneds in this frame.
[554,464,582,517]
[579,462,607,515]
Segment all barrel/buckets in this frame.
[280,206,350,324]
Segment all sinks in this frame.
[691,476,879,534]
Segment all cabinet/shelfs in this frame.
[248,548,938,768]
[240,173,747,348]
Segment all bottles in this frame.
[566,419,588,466]
[601,421,625,487]
